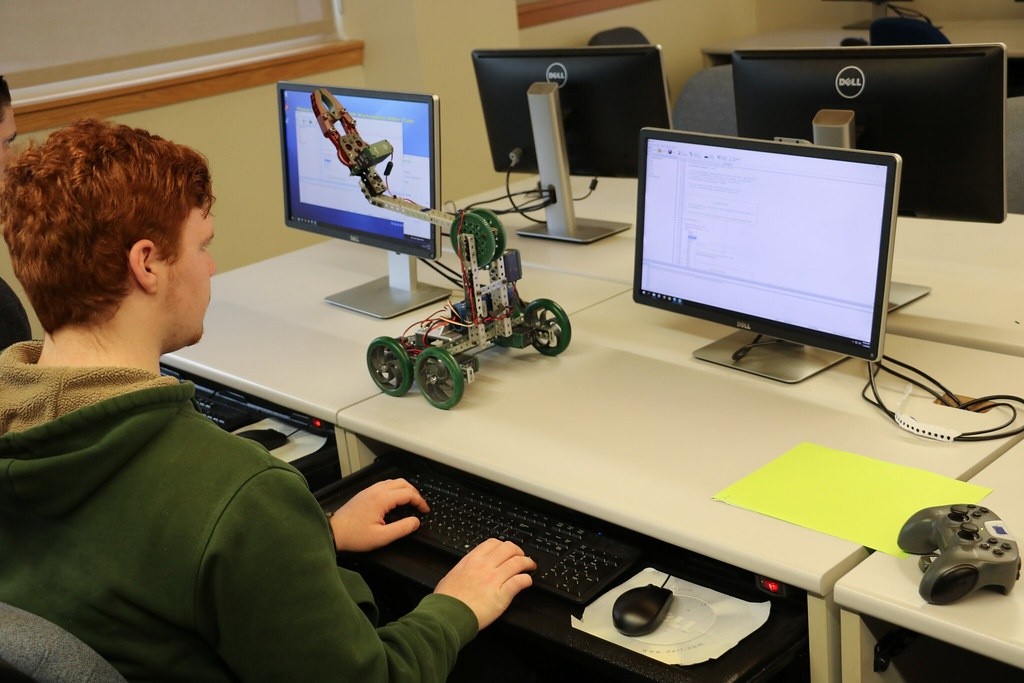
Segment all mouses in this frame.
[612,583,674,637]
[235,428,287,452]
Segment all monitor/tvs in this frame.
[276,81,442,261]
[633,45,1008,361]
[471,45,672,178]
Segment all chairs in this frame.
[669,64,734,138]
[0,604,125,682]
[1006,96,1023,214]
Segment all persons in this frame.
[0,75,536,683]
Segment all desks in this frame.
[704,17,1024,59]
[158,177,1024,683]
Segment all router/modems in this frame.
[892,395,1008,444]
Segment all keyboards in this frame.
[351,464,646,607]
[159,363,269,433]
